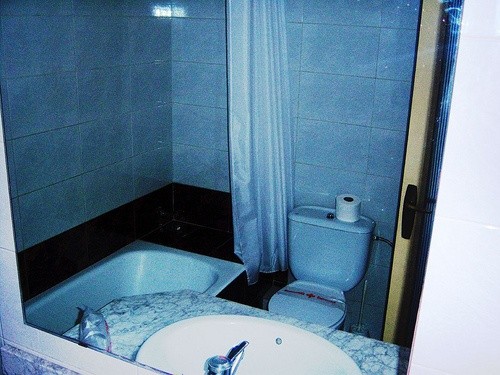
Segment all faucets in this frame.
[208,339,249,375]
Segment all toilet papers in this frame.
[335,192,362,221]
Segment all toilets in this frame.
[267,204,376,330]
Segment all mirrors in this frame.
[1,1,465,375]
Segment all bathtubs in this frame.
[22,239,247,337]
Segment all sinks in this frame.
[136,314,362,375]
